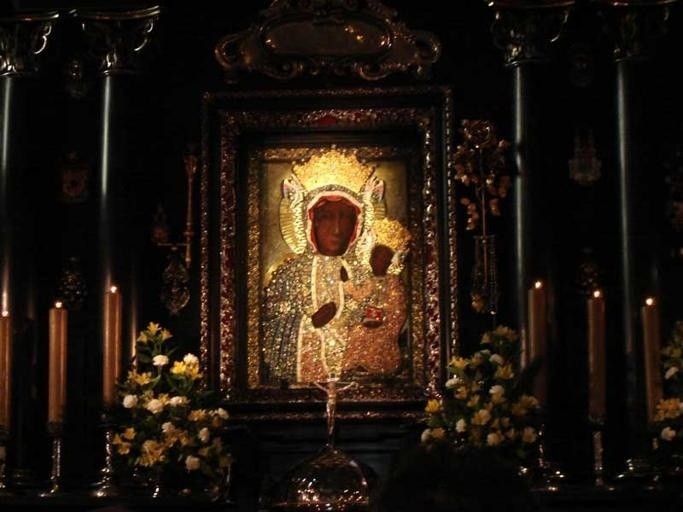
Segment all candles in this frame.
[0,288,124,431]
[529,289,664,426]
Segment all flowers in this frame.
[87,318,231,485]
[417,324,542,460]
[655,318,683,456]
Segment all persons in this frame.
[312,380,354,434]
[338,245,413,377]
[258,201,411,379]
